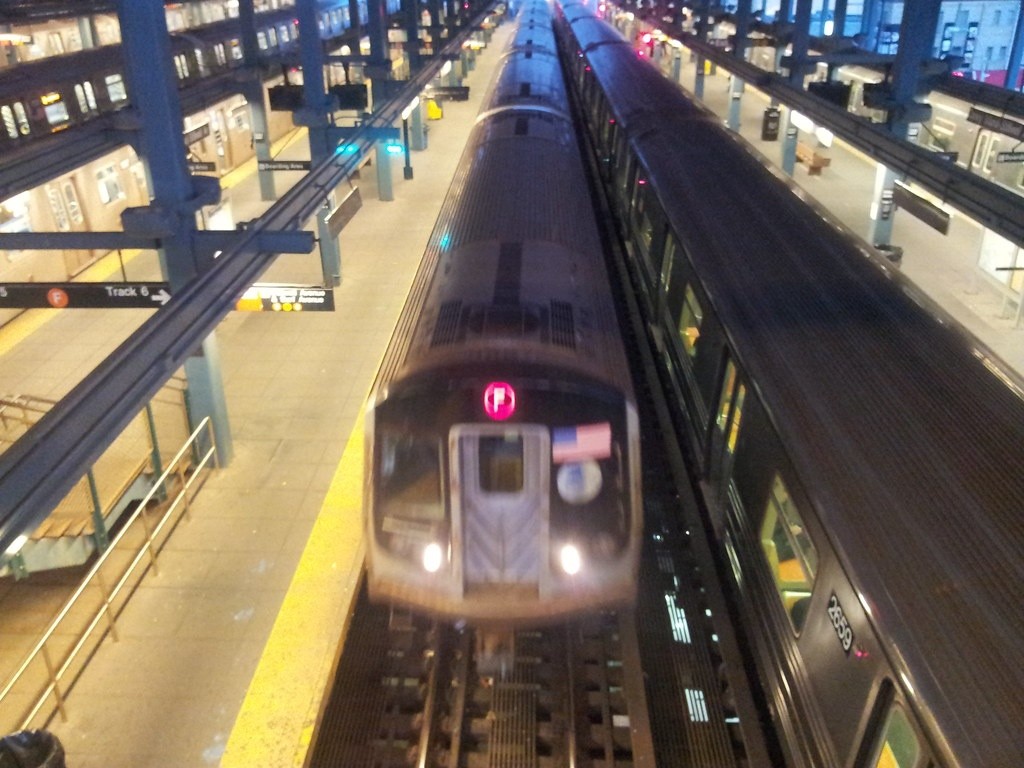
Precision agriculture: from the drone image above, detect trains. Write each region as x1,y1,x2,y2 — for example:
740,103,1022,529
361,0,644,631
548,0,1023,768
